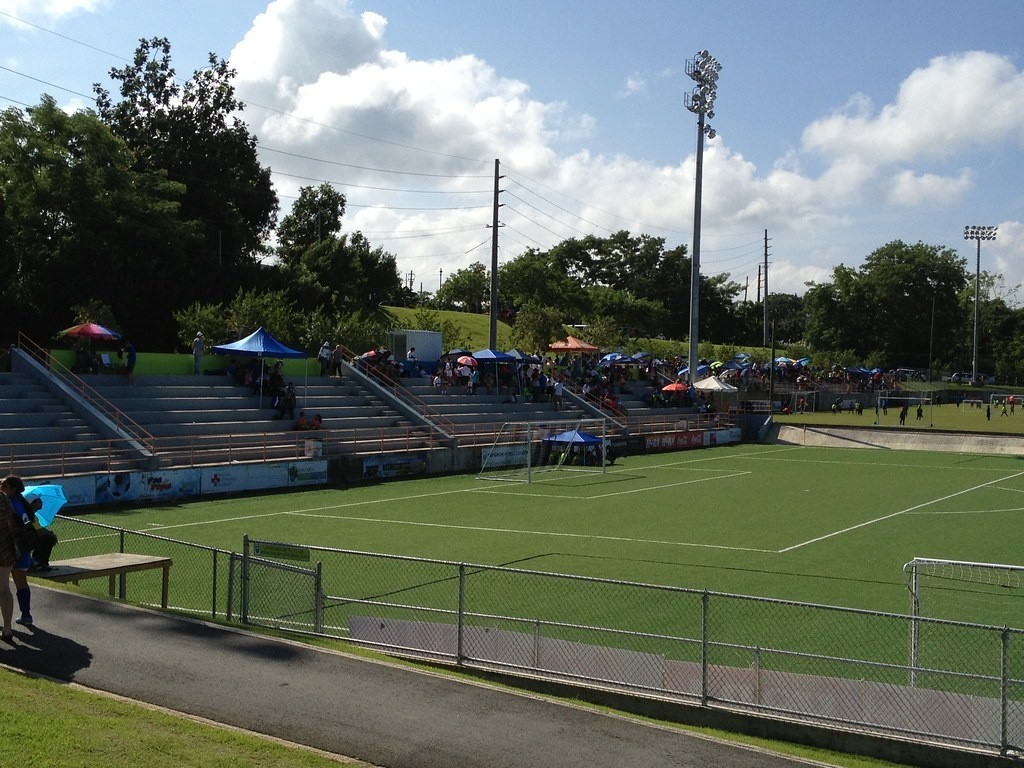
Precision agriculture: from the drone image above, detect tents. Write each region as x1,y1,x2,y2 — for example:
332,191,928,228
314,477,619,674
211,327,308,408
472,348,544,396
692,375,738,409
543,429,603,466
549,335,598,351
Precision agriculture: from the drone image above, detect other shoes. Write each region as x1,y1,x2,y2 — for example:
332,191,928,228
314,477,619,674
0,629,13,642
17,616,31,625
472,393,479,396
30,564,54,572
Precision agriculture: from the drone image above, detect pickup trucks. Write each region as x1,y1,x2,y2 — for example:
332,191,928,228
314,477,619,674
882,368,926,382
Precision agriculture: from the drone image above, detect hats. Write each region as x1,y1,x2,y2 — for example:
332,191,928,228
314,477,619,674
323,341,329,347
197,332,203,337
24,492,42,504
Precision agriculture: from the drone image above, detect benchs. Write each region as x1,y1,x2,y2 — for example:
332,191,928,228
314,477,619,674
0,364,894,468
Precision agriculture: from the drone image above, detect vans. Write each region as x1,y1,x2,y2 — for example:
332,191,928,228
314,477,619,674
951,372,996,385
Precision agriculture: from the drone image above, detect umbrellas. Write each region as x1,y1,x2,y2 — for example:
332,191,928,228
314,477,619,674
440,348,472,361
843,367,883,373
456,356,478,365
600,352,650,365
662,382,688,390
21,483,68,528
60,322,121,340
679,352,810,377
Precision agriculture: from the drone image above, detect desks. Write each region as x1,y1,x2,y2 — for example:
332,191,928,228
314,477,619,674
27,552,173,608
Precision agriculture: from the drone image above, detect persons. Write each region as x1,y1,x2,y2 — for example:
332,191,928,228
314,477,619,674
370,347,433,386
434,354,842,415
317,341,343,377
986,394,1014,421
0,477,58,641
848,400,863,415
191,332,206,375
227,359,322,430
547,444,616,467
956,398,960,408
847,376,886,393
910,370,921,381
547,352,580,366
125,341,137,386
882,399,923,425
936,394,942,406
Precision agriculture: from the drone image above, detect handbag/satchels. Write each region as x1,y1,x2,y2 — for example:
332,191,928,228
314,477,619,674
13,519,40,554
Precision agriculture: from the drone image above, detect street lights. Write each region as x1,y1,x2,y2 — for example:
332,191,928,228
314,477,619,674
964,226,998,382
683,49,723,384
